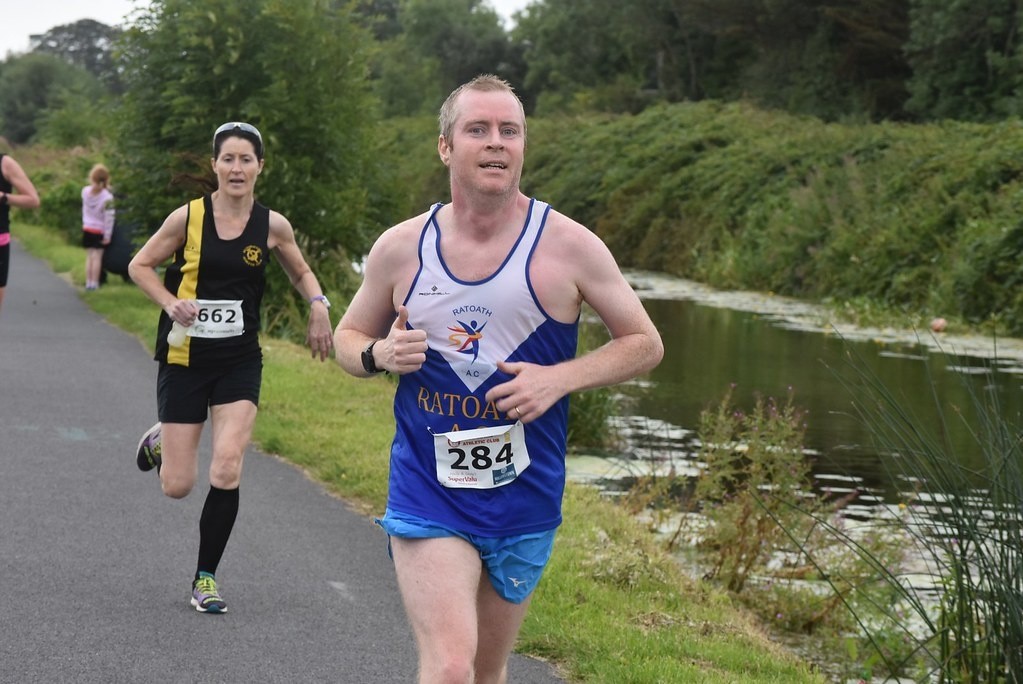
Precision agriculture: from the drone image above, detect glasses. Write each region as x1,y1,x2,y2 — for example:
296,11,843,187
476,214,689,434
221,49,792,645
213,122,263,152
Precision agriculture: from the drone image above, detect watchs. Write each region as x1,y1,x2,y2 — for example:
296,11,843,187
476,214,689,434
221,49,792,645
361,339,390,375
1,193,7,202
310,295,331,307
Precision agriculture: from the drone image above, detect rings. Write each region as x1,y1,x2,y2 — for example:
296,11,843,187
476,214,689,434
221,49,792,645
515,406,521,415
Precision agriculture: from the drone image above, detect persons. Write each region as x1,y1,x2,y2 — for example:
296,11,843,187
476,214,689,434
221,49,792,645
99,192,138,284
0,153,40,306
80,164,115,290
331,76,664,684
127,122,334,612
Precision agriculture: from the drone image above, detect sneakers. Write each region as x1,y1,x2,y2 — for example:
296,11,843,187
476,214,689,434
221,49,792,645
190,571,227,613
136,422,162,472
